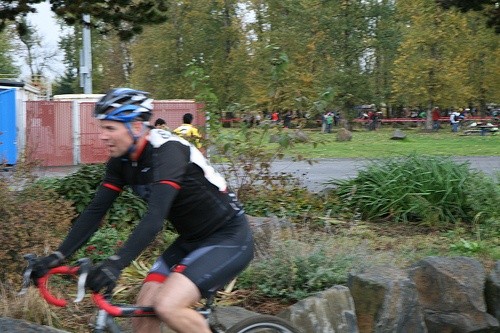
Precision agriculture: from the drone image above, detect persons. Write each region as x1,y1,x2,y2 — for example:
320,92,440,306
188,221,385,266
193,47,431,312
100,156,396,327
22,88,253,333
256,105,500,137
152,113,202,153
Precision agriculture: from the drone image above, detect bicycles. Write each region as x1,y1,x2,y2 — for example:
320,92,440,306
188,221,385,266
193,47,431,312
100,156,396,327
20,252,302,332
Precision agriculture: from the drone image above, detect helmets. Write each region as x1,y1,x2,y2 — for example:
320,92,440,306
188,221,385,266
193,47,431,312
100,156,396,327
92,87,154,122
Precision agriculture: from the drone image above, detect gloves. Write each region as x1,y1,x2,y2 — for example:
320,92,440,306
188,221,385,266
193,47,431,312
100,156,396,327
84,254,127,295
21,254,63,287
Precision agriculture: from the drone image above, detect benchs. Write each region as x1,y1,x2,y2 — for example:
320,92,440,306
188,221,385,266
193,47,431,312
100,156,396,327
478,126,500,135
